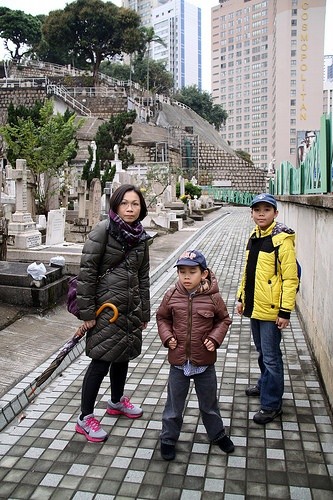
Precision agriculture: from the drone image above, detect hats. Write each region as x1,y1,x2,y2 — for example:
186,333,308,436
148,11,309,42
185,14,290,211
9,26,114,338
173,249,207,270
250,193,277,210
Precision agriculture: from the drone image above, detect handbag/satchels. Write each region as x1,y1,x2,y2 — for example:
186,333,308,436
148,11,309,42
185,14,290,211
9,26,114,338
67,276,98,319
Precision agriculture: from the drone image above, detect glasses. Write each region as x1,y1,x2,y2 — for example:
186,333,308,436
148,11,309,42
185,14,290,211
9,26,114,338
120,201,141,211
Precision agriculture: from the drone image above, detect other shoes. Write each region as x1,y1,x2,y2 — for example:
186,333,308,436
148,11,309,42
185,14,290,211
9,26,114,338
253,408,283,424
160,442,176,461
216,436,235,453
245,387,261,396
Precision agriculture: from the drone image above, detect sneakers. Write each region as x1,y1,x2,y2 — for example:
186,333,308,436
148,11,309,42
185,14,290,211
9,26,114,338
75,412,107,442
106,395,143,418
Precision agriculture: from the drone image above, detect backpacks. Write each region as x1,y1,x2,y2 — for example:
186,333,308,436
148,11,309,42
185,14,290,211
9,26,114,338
274,247,302,295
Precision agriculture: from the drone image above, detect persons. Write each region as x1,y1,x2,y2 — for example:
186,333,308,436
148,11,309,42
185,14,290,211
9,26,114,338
156,250,236,460
236,193,299,421
75,184,151,441
298,131,316,164
